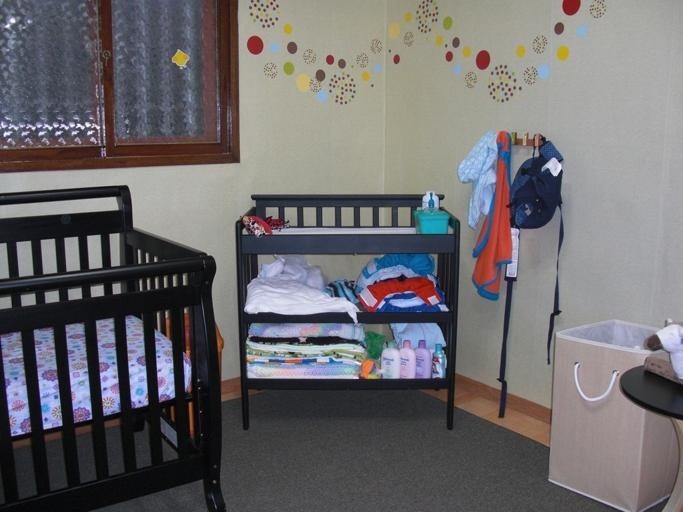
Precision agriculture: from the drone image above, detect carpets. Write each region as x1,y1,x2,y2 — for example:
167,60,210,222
0,389,681,512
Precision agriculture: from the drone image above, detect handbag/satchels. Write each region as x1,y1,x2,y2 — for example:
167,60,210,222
512,134,563,228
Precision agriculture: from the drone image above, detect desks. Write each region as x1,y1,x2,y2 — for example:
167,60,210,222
620,364,683,512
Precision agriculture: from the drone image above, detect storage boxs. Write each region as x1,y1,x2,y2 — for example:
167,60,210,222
543,317,682,512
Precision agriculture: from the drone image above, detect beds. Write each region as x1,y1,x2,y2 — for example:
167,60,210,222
0,184,228,512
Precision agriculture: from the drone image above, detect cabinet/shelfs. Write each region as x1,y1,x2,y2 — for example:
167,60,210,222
233,191,462,433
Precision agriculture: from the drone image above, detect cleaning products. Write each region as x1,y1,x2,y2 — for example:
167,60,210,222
423,190,440,208
